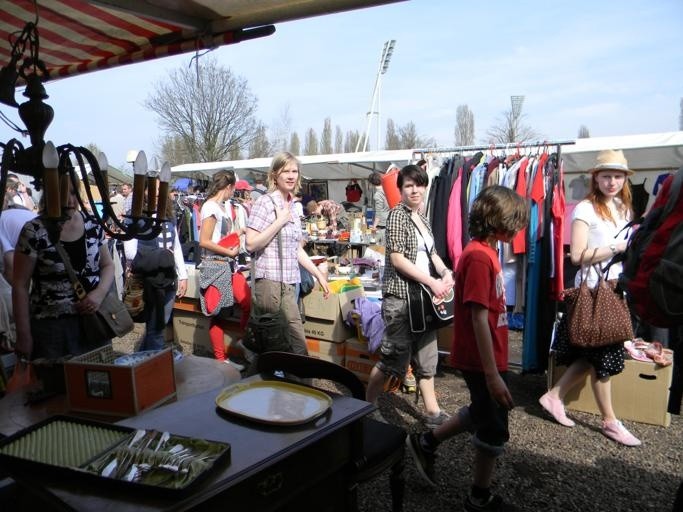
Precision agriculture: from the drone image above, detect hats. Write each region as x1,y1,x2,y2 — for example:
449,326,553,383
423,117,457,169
585,149,633,175
235,180,253,190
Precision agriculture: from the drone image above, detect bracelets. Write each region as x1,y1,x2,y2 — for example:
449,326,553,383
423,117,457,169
608,243,619,255
439,269,454,277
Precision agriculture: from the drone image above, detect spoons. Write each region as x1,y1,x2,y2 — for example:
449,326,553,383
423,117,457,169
100,427,215,483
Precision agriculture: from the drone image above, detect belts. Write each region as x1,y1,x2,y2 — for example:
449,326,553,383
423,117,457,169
376,226,385,229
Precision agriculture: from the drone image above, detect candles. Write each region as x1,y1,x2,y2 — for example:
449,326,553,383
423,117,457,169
132,151,148,217
147,155,159,212
41,138,63,218
98,153,111,202
156,160,171,220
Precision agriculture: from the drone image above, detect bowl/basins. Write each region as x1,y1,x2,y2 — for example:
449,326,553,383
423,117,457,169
359,277,375,287
337,266,351,274
317,233,326,239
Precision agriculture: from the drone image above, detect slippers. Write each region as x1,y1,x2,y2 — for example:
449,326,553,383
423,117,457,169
622,337,673,366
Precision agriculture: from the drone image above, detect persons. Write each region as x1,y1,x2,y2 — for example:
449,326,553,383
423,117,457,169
538,150,644,446
365,165,455,426
370,172,389,229
405,185,521,512
0,151,350,386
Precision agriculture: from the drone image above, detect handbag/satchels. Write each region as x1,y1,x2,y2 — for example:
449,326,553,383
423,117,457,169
242,311,291,353
87,293,135,341
0,353,18,392
298,262,314,292
563,247,633,349
406,269,457,333
122,276,145,317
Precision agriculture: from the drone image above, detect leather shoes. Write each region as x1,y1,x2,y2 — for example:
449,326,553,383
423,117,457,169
224,358,245,371
236,339,256,363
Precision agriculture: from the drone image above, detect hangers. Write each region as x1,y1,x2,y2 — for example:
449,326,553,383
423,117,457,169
416,139,551,158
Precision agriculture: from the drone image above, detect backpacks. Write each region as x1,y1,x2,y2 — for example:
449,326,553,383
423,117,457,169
603,172,682,329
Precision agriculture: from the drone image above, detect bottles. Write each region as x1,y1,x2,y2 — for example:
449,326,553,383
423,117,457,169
369,229,376,245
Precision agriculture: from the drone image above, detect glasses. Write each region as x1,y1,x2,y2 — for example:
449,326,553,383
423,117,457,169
224,170,234,175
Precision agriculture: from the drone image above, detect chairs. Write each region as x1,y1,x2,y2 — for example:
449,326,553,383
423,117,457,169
251,350,405,511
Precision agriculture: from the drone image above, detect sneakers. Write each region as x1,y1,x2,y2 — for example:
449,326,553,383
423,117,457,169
600,421,640,446
366,408,389,424
422,410,452,428
538,393,575,427
401,365,415,387
463,493,524,511
406,433,437,486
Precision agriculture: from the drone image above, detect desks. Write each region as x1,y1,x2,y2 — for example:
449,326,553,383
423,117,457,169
1,357,406,511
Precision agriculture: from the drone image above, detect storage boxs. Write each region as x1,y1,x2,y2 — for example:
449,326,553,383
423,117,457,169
553,348,674,426
62,342,177,418
171,264,416,388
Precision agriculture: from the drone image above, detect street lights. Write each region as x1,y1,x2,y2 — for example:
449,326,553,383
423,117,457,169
346,36,398,154
